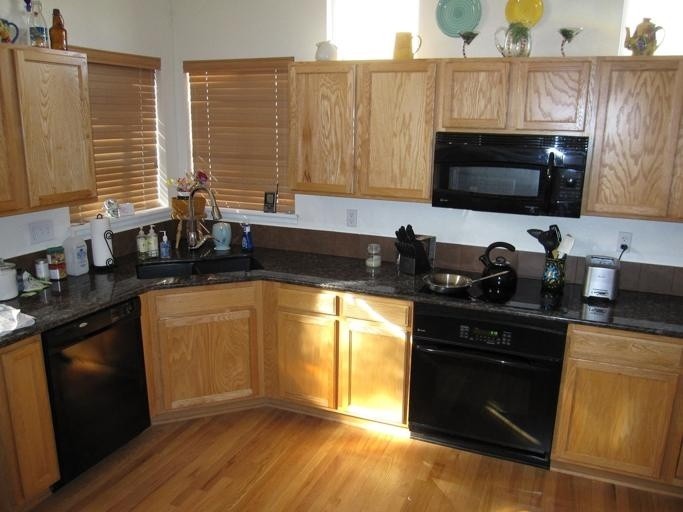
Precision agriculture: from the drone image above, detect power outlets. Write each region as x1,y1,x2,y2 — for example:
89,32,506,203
617,232,633,253
346,208,357,227
28,219,55,245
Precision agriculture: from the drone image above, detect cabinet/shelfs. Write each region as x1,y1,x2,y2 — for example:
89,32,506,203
274,281,411,441
287,58,438,204
152,281,267,426
441,56,592,131
0,332,61,512
0,42,98,217
580,55,683,223
549,323,683,499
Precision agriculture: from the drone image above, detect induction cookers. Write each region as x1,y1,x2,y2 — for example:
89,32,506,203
420,267,546,312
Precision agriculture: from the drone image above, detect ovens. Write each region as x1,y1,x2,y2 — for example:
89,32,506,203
408,340,563,470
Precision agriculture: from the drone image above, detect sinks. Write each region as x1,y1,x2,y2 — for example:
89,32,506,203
194,255,265,275
135,260,201,280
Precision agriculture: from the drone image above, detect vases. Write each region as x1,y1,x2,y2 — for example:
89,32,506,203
170,192,208,219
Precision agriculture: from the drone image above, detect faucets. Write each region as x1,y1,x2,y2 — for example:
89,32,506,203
185,186,223,250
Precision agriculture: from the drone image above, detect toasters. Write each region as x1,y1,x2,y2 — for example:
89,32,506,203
581,301,615,324
582,255,621,301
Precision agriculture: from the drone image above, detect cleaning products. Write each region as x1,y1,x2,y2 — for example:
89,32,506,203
241,215,253,252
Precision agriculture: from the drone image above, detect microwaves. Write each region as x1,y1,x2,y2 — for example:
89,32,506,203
429,131,588,218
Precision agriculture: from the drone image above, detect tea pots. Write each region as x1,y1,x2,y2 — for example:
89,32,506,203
480,241,518,292
624,17,664,55
492,22,533,61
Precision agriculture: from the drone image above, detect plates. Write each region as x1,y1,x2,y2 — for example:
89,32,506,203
505,0,544,30
423,274,473,293
436,1,482,38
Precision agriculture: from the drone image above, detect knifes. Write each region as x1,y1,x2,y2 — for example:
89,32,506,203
393,224,416,243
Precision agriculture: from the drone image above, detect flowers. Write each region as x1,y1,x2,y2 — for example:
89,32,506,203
163,167,209,192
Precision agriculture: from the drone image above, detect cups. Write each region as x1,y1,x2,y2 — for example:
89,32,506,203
394,31,422,60
1,20,20,44
315,42,339,61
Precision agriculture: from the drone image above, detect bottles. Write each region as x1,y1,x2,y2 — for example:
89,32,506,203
366,268,382,281
365,244,382,268
39,274,90,304
35,246,66,281
27,2,70,50
541,291,564,312
241,223,254,251
541,256,565,289
212,222,232,250
0,258,18,302
